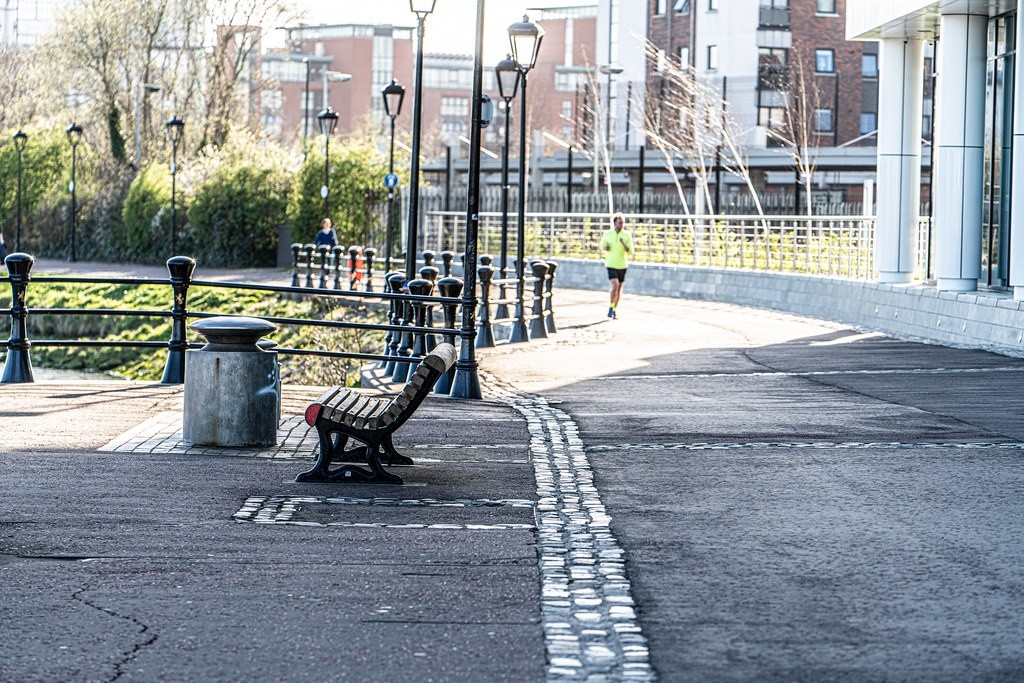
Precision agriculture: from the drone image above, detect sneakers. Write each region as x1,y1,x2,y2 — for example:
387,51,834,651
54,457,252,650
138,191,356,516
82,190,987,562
608,307,617,319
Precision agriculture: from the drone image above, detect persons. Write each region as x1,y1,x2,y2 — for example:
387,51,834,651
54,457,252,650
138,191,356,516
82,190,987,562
599,217,634,318
314,218,339,274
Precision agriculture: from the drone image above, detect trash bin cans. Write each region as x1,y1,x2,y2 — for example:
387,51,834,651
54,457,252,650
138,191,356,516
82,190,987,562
183,317,282,447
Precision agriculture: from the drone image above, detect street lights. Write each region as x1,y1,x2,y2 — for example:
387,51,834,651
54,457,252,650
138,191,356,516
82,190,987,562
66,123,85,262
381,77,406,272
504,13,547,320
165,113,186,258
496,52,522,320
403,0,438,278
318,106,341,219
13,130,28,253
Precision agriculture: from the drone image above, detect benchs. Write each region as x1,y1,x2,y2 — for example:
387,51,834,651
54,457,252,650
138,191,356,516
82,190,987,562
295,342,457,485
